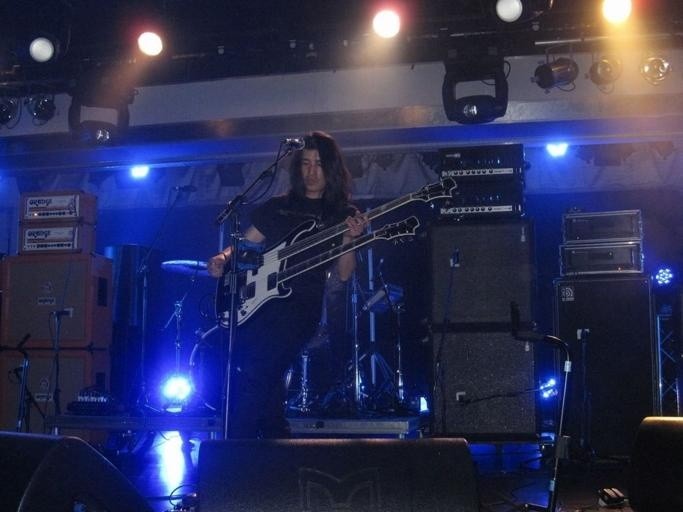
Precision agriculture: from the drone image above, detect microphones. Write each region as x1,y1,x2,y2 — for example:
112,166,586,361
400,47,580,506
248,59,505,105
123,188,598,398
510,301,520,334
283,136,306,151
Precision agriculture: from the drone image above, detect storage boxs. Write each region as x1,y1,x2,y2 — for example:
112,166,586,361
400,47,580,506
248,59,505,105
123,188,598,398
18,225,97,251
19,188,97,224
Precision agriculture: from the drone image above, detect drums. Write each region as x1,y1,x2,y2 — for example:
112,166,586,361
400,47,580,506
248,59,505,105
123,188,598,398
189,317,293,415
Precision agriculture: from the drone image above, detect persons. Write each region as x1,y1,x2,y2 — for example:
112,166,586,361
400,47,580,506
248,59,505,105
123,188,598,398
207,130,371,439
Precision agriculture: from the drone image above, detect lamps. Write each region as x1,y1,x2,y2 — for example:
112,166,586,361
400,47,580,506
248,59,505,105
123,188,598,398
0,68,60,128
216,161,244,186
441,36,515,125
59,67,141,149
531,32,677,94
345,155,363,179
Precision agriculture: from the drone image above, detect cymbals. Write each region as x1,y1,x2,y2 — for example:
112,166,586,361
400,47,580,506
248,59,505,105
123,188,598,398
161,261,212,277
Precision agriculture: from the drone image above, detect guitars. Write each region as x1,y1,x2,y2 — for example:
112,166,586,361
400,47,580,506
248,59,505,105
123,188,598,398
216,179,457,328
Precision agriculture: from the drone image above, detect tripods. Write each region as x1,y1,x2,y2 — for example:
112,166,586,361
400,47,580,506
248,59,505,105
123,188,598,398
285,207,398,417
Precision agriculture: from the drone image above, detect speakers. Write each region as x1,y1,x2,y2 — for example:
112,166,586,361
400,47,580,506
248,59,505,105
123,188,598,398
195,438,483,511
553,278,663,436
2,253,115,350
627,416,683,512
423,220,536,331
429,328,539,437
0,430,154,512
0,349,112,443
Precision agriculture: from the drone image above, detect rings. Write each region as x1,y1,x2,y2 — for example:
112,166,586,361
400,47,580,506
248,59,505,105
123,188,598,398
211,262,215,265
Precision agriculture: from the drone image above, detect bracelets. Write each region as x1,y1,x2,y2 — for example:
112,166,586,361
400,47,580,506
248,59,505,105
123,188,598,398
217,252,227,261
